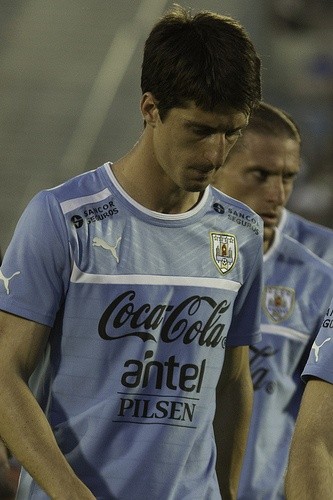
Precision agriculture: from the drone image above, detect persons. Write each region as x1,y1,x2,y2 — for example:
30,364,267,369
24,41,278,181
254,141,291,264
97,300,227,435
0,2,268,500
277,206,333,267
211,102,332,500
